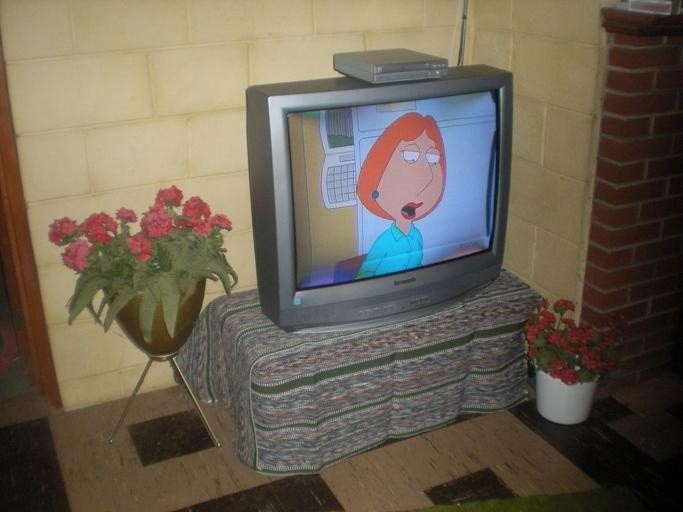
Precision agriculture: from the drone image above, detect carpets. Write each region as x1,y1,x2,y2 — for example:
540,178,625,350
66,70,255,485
406,487,644,511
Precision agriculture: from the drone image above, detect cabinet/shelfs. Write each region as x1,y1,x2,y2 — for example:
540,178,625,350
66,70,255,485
171,267,544,478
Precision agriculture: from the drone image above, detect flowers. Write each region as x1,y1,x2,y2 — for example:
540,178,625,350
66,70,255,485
523,298,632,384
45,185,238,345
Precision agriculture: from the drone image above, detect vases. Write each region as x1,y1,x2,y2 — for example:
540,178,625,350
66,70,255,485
533,354,599,427
102,277,205,362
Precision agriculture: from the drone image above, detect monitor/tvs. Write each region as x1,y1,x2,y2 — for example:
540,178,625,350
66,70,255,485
245,64,514,334
